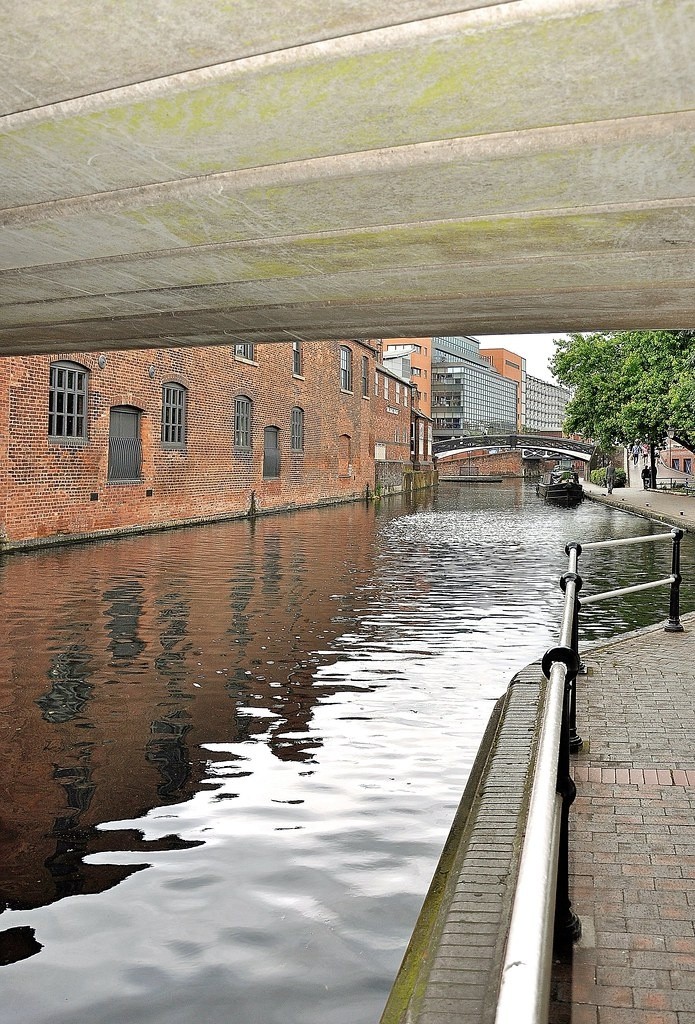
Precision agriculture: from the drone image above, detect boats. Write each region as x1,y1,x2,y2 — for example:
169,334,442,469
537,469,584,506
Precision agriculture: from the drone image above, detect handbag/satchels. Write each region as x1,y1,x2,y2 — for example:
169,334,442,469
631,453,633,460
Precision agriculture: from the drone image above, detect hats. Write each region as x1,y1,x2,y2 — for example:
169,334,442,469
609,461,614,464
644,444,647,448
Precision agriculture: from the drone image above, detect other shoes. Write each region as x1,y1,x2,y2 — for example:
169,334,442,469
610,491,612,494
608,489,611,493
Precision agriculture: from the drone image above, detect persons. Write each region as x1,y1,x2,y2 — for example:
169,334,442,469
641,442,649,466
632,441,639,465
605,459,618,495
571,465,575,472
641,465,657,489
